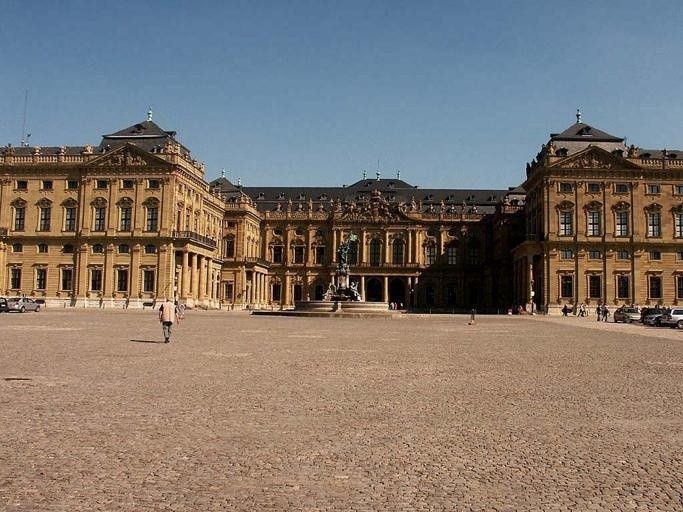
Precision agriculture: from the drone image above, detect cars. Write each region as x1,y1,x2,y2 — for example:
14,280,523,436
7,296,41,313
613,306,641,324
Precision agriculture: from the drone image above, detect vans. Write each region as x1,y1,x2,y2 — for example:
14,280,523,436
1,297,8,313
640,304,683,329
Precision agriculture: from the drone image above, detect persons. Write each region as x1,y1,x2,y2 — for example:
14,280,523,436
159,298,179,343
338,241,350,268
562,301,672,322
390,302,403,310
350,281,360,301
